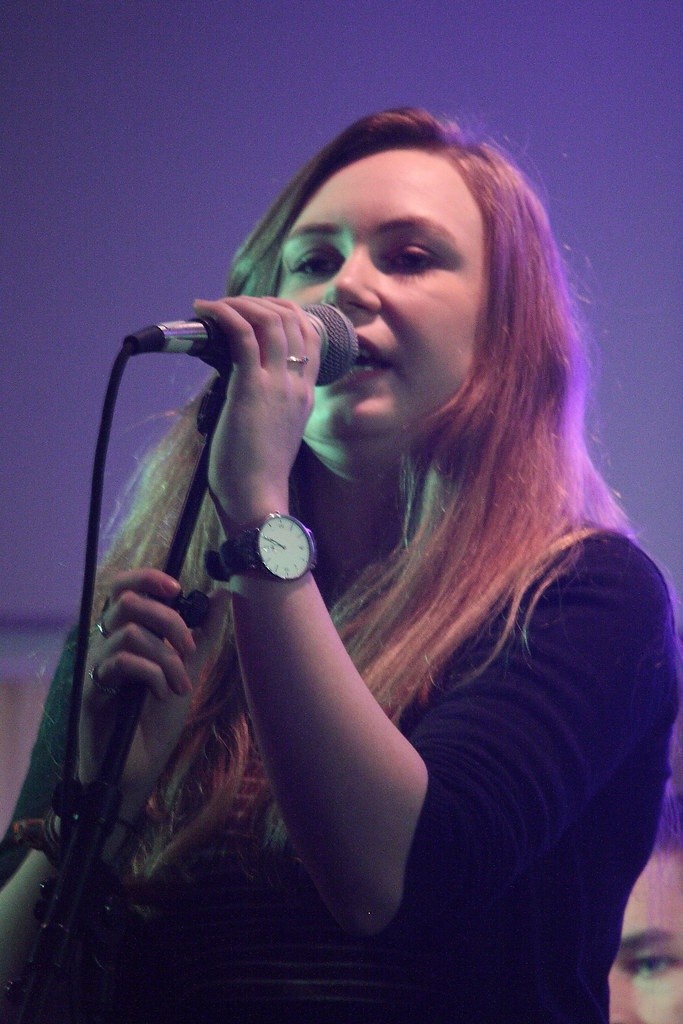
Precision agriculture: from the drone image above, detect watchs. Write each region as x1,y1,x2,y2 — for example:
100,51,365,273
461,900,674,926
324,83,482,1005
204,511,321,582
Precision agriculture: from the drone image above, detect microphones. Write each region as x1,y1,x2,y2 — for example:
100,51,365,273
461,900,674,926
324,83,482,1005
124,304,358,385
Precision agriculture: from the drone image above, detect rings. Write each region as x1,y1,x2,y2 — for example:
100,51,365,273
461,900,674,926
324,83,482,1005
96,617,109,639
287,357,309,364
87,662,119,695
100,596,109,613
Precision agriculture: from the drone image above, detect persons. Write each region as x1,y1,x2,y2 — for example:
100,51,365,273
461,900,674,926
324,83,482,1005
0,105,683,1024
